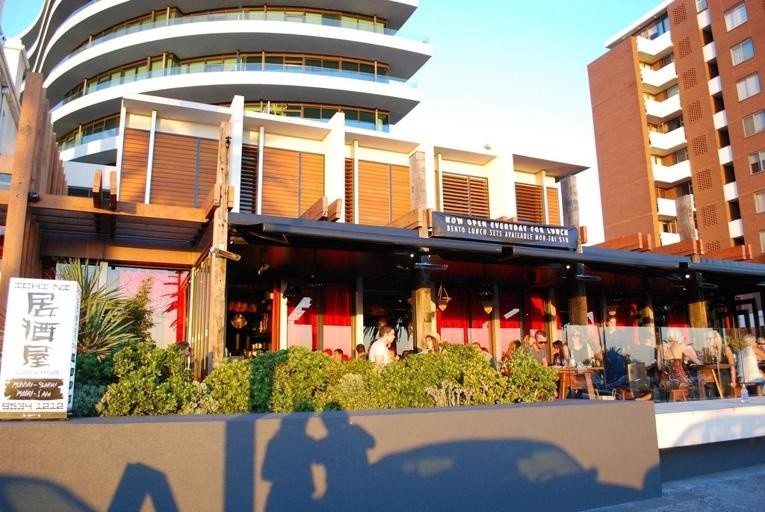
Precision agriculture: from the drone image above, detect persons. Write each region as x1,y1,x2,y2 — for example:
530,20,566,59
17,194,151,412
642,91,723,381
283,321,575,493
312,320,438,372
502,328,593,374
176,341,193,360
473,341,497,370
606,318,765,401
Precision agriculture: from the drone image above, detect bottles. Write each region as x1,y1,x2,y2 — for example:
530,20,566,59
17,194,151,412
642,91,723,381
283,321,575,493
703,347,719,365
590,356,601,367
739,384,749,404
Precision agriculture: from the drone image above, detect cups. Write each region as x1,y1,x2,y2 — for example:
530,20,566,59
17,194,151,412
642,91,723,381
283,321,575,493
555,359,560,366
567,356,577,369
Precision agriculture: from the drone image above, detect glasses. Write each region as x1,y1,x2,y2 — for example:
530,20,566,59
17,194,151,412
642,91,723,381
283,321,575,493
539,341,547,343
